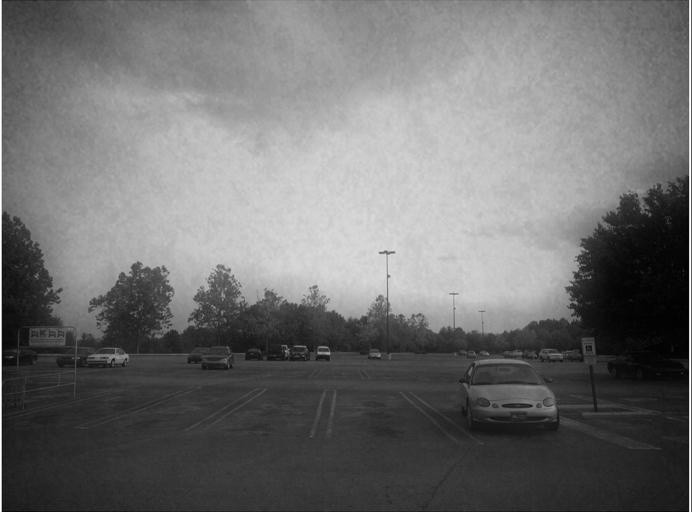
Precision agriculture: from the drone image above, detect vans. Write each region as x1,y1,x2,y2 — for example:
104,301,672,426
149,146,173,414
316,345,332,361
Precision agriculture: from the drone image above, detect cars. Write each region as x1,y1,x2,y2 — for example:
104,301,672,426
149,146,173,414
185,343,309,370
86,346,131,368
2,345,40,367
459,347,585,363
455,357,564,434
367,348,383,360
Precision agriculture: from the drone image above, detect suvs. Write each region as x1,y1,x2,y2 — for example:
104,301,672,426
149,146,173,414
55,346,97,367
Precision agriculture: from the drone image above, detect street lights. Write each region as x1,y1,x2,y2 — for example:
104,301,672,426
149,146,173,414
378,249,398,354
447,291,460,353
477,309,487,337
